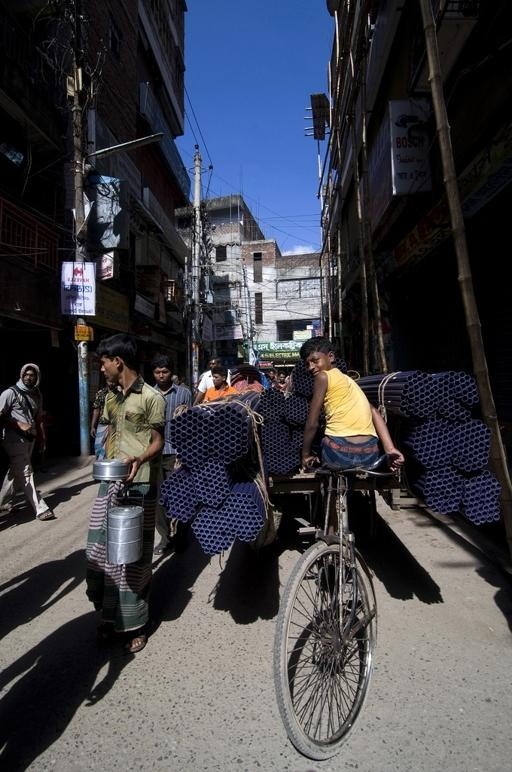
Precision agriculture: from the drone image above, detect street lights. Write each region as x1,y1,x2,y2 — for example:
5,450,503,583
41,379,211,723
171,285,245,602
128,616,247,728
69,131,167,457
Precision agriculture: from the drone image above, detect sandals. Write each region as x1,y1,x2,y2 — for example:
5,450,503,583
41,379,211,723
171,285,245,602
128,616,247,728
36,510,54,520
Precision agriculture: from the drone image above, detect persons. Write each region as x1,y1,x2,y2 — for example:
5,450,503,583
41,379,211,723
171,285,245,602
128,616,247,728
0,363,56,519
90,354,293,553
300,335,405,592
84,331,169,655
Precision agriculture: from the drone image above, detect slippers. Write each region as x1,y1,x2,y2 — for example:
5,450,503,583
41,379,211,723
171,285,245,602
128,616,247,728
126,634,146,654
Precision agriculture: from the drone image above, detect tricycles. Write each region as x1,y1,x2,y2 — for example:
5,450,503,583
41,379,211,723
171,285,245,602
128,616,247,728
271,453,407,761
